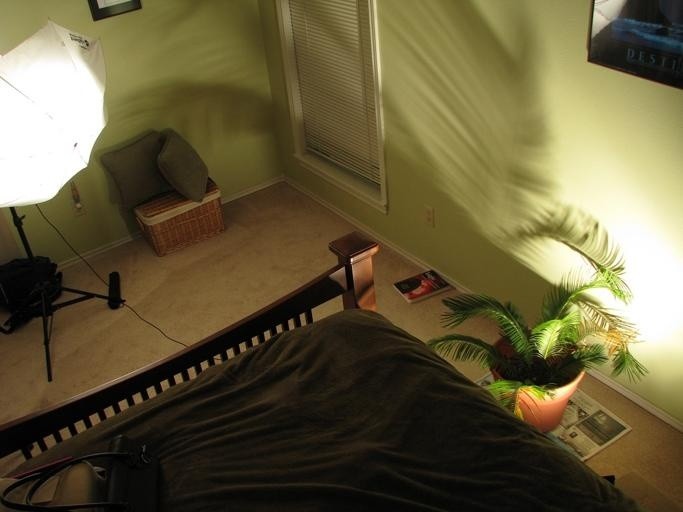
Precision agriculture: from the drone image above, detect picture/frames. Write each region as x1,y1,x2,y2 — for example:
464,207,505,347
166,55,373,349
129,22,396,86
88,0,142,22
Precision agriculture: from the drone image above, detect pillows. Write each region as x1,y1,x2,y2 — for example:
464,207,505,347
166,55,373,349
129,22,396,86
102,132,175,211
156,129,208,202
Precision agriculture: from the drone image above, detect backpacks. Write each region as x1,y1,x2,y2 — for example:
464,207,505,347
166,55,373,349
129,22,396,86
0,255,62,315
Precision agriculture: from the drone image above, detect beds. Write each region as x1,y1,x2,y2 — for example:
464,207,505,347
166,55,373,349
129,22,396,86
0,232,641,512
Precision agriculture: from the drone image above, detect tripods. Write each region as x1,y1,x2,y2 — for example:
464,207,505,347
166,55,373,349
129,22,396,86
0,207,108,381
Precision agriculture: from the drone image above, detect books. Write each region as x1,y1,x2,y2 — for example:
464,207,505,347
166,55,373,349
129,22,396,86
393,269,453,304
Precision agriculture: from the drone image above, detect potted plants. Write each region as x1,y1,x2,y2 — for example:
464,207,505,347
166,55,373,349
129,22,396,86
425,264,649,433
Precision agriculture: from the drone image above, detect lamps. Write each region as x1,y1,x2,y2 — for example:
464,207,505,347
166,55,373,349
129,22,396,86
0,16,110,208
